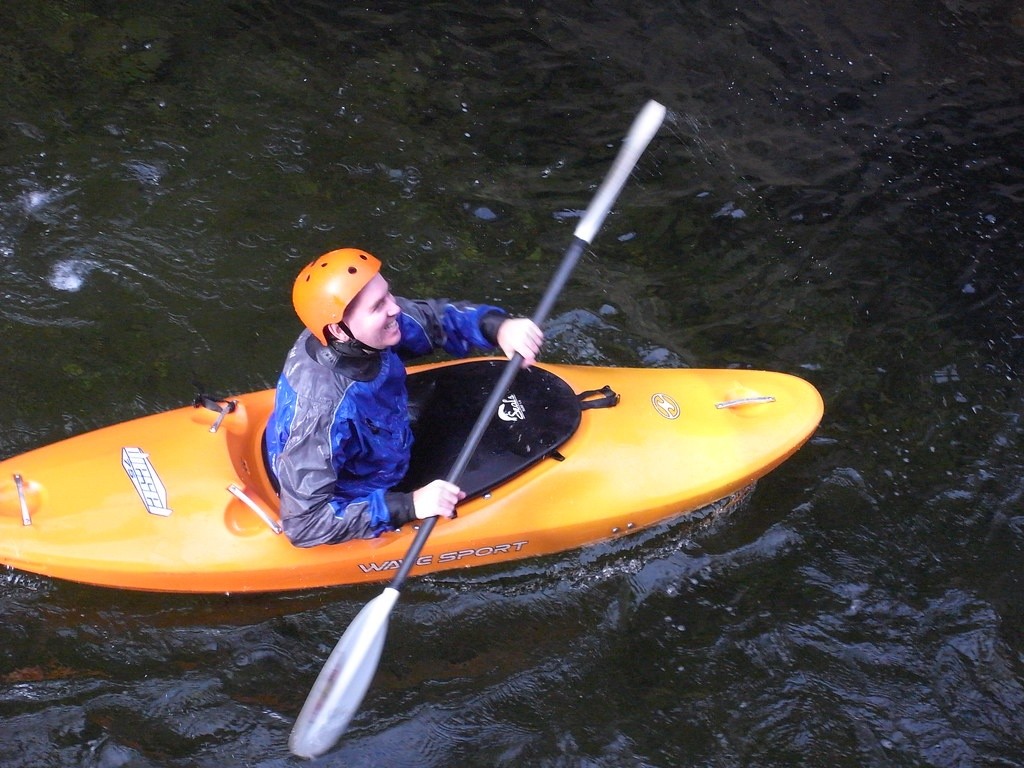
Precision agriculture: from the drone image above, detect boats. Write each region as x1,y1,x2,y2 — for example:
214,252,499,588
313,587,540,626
1,353,826,592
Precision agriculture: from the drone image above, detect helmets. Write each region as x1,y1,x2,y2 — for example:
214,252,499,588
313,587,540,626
293,249,381,346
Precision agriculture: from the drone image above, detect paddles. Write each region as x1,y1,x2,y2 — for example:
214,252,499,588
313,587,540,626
281,93,684,761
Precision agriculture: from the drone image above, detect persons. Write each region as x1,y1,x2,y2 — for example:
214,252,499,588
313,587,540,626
266,248,544,548
